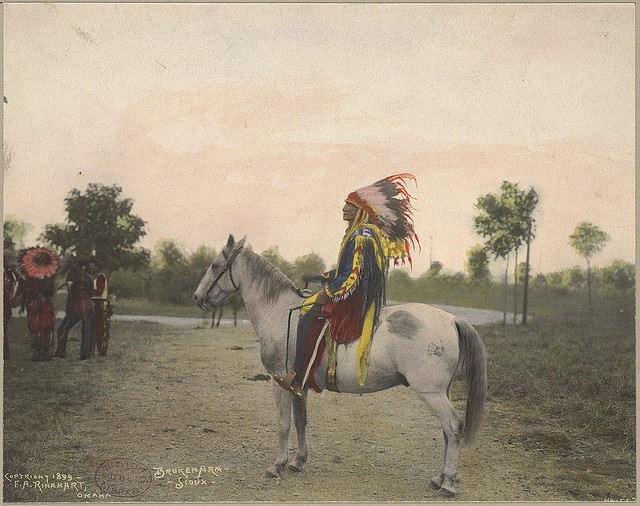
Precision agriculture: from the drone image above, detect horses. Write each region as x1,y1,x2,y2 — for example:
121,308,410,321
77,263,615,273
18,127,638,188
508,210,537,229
192,234,489,499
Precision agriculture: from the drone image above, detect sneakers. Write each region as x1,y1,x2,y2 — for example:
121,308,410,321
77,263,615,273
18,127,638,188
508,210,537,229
272,373,307,400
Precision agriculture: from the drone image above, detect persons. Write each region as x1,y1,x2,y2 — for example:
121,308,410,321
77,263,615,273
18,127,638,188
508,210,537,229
90,258,111,357
4,253,25,359
18,249,56,361
286,171,423,395
54,256,92,360
